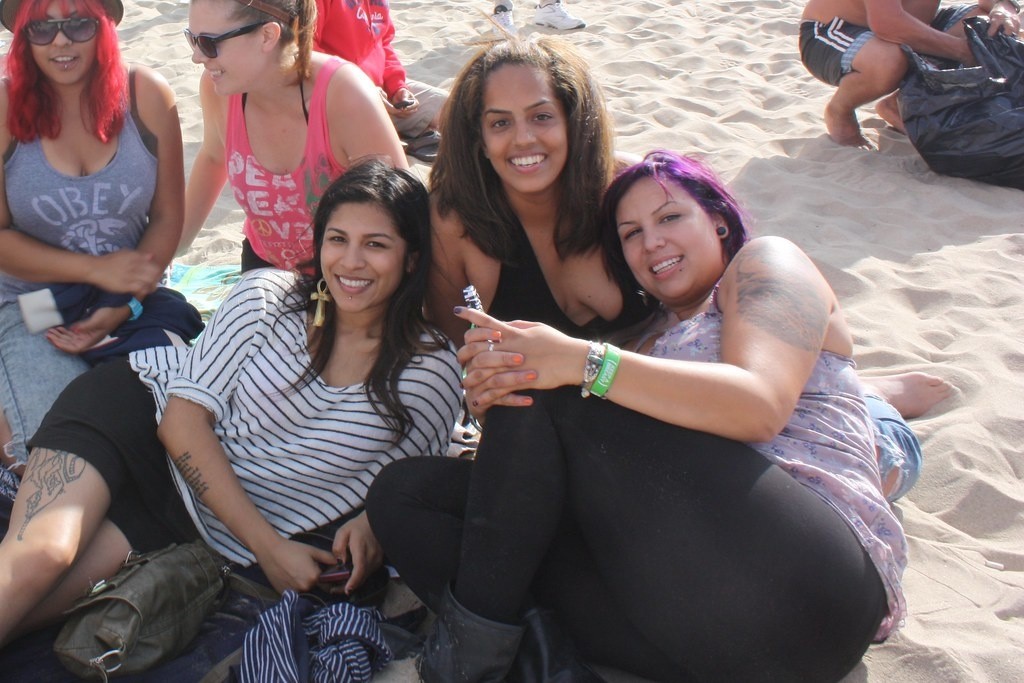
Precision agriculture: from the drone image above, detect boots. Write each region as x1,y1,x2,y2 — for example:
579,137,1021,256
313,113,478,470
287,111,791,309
377,577,529,683
507,609,607,683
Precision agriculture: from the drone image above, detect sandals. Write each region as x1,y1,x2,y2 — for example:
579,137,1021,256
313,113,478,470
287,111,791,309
398,130,442,162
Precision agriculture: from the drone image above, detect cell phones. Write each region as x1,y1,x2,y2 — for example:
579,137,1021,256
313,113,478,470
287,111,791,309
394,100,414,109
319,555,353,581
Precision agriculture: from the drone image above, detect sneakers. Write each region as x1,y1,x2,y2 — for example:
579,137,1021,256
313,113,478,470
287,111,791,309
534,0,586,30
490,0,515,38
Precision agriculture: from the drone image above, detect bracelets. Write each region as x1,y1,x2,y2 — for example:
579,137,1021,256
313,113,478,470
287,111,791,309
127,297,144,321
581,341,621,398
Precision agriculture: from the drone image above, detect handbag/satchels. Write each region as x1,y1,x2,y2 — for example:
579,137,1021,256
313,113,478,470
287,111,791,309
54,539,231,682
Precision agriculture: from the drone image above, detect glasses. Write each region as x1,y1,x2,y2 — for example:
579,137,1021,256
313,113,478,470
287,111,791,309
22,18,99,45
183,19,284,58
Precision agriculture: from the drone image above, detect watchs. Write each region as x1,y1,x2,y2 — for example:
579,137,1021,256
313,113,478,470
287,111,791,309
1000,0,1020,13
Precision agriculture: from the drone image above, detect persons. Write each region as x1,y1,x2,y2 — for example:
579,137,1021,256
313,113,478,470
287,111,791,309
423,32,962,504
367,151,909,683
493,0,586,34
313,0,450,161
0,0,185,476
0,162,463,649
172,1,409,275
798,0,1024,152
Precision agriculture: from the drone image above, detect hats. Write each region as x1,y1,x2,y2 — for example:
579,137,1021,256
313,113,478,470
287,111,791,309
0,0,124,34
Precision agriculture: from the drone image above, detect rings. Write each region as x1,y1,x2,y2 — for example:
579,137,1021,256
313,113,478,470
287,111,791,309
488,340,494,351
